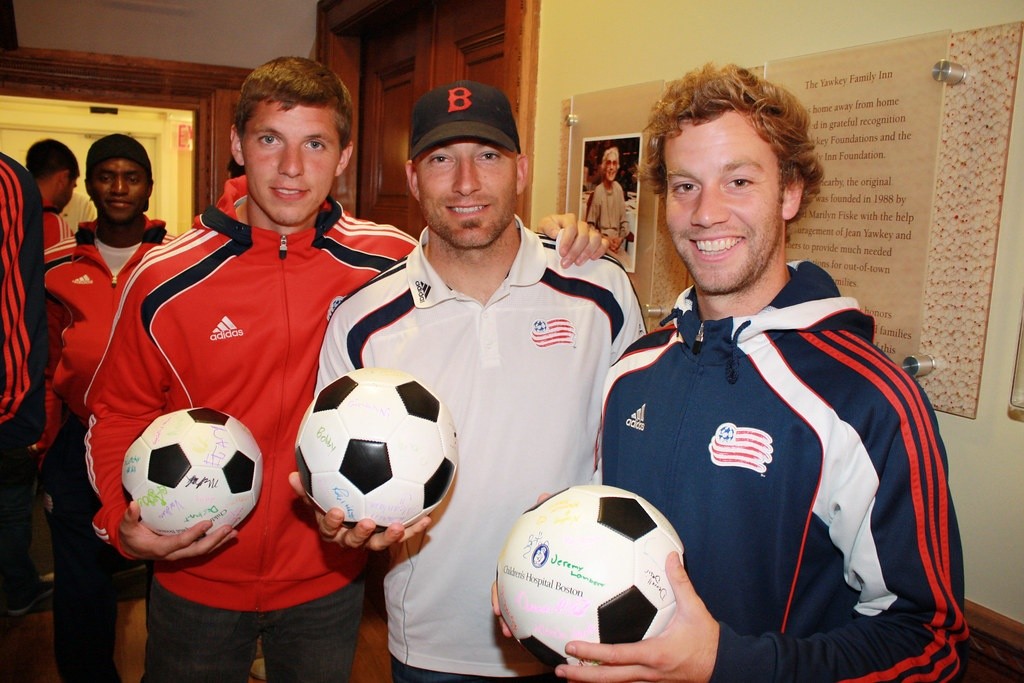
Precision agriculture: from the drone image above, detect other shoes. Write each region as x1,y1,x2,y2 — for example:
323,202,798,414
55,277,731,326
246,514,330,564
3,570,54,614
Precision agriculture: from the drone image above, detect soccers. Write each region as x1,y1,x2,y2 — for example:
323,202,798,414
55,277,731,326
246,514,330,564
495,484,688,667
123,404,265,536
295,370,458,531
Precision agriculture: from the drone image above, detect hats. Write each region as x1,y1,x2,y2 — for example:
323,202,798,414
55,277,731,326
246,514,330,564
409,80,521,156
86,134,152,211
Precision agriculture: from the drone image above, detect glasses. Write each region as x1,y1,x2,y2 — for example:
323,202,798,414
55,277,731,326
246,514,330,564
605,160,618,166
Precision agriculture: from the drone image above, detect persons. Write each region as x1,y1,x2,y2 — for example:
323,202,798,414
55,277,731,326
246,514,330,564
587,146,630,252
0,135,176,683
288,80,649,683
86,55,610,683
494,64,969,683
